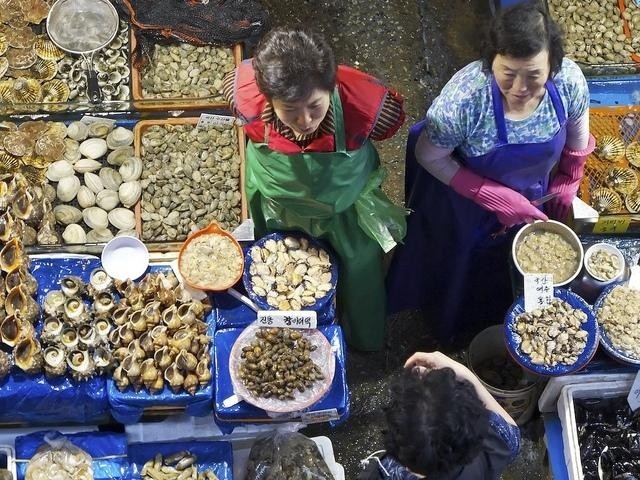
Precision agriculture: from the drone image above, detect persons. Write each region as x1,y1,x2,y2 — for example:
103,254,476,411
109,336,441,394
357,351,521,479
221,27,409,350
385,6,596,344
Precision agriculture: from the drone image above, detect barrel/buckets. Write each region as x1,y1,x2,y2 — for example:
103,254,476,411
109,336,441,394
466,323,545,427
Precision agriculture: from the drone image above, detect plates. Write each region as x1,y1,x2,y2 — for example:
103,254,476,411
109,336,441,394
583,244,625,282
501,287,600,376
241,231,339,318
590,273,640,366
228,317,337,414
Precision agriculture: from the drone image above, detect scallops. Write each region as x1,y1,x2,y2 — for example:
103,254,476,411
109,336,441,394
0,1,71,179
591,135,640,215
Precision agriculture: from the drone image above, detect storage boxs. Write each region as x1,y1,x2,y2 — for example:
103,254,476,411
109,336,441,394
536,0,640,73
557,378,640,480
132,117,249,241
129,16,244,117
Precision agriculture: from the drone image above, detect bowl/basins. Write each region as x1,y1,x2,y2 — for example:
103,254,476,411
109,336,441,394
100,236,150,281
509,218,584,289
178,223,245,292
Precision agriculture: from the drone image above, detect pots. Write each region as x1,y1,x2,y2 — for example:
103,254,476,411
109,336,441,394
46,0,120,104
